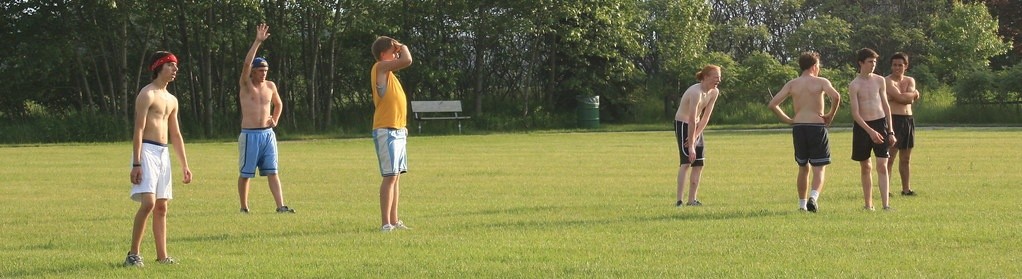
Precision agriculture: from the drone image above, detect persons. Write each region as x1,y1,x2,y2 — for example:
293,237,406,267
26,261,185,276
238,23,297,214
674,66,721,208
122,51,192,268
849,47,898,212
768,52,840,213
884,51,919,197
371,36,415,232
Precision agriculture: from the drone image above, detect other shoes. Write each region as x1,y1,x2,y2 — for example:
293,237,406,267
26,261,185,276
901,190,916,196
806,197,818,212
687,200,701,205
276,206,295,213
394,221,408,229
382,224,396,231
240,208,249,213
676,200,683,207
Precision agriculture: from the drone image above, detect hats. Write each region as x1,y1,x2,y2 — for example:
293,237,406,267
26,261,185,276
252,58,269,68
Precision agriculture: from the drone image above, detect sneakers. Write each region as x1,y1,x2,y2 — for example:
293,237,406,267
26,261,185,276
123,251,144,267
155,256,176,265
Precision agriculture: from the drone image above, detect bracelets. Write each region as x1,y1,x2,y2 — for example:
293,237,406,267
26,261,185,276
132,164,141,167
400,44,404,50
887,131,894,135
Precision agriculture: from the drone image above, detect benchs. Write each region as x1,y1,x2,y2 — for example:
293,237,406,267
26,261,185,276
411,100,470,136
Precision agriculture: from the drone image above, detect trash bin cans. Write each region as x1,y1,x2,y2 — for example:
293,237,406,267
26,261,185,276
576,94,600,129
